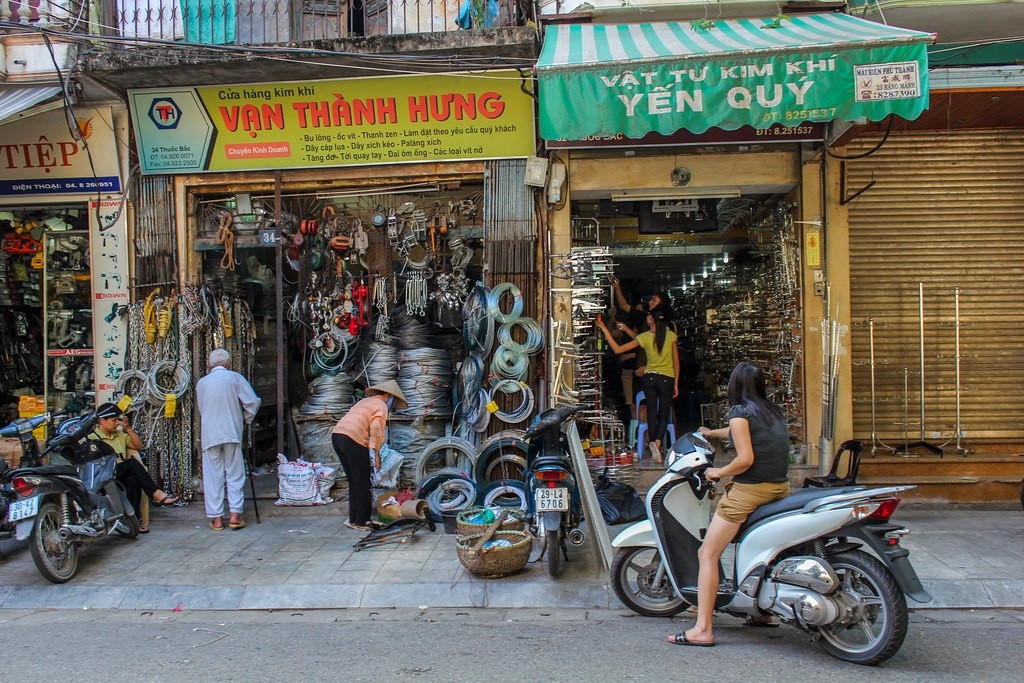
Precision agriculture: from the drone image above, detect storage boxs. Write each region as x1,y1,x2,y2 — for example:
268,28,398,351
19,412,46,440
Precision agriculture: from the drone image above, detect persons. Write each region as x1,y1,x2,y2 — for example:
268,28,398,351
667,362,788,646
87,402,179,533
595,275,679,464
331,379,409,528
195,348,261,530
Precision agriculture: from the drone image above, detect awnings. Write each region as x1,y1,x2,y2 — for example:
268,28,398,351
532,11,938,141
0,84,64,120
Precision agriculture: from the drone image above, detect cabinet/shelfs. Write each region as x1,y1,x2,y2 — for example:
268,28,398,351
42,230,95,459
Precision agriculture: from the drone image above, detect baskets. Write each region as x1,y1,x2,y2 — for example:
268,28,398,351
456,530,532,578
456,507,527,535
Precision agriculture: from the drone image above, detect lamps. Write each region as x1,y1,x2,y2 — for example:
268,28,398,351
611,187,744,201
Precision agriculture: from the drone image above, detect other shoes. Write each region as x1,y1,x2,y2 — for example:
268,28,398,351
153,494,180,506
351,519,383,528
139,525,150,533
649,442,663,463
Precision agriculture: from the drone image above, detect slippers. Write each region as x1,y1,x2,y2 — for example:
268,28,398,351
667,632,714,646
209,520,224,531
229,517,245,528
742,616,779,627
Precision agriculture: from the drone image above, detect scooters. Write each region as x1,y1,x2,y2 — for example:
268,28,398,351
519,401,595,578
610,426,933,666
0,401,143,583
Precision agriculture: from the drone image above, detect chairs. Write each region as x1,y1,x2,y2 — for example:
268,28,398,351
636,391,676,460
804,439,866,538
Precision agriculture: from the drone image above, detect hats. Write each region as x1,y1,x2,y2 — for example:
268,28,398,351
365,380,409,409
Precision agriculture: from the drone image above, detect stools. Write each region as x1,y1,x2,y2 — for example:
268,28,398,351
628,419,640,449
